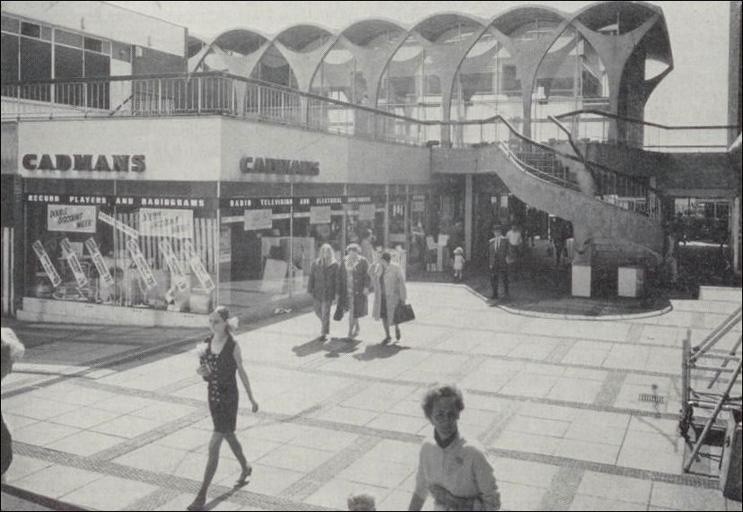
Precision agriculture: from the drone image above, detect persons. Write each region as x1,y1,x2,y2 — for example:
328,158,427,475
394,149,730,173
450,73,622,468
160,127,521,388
452,246,466,279
408,385,501,512
1,326,26,478
489,224,511,299
346,490,377,511
423,231,438,272
506,224,523,274
373,252,405,345
187,306,259,512
306,242,339,340
337,243,371,343
547,214,570,263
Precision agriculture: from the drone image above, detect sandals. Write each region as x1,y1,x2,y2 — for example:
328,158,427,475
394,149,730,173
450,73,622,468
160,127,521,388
185,497,205,512
233,467,252,483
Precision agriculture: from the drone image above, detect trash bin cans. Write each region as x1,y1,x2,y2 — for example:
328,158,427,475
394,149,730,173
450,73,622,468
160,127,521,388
617,265,645,298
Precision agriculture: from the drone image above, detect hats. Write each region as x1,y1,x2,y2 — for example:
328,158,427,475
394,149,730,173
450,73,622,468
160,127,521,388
381,252,392,259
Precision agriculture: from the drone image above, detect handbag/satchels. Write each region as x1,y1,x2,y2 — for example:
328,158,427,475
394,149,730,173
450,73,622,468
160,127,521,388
352,294,368,319
332,298,345,321
389,300,415,326
